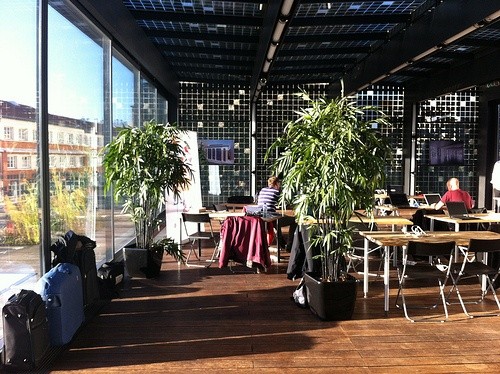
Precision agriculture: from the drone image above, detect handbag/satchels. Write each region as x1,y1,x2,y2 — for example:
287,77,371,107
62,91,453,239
96,261,124,291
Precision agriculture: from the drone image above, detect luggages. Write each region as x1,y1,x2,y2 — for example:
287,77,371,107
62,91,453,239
34,262,86,349
3,288,54,374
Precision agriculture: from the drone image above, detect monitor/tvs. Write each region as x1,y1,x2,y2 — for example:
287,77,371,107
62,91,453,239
198,139,234,164
421,140,464,166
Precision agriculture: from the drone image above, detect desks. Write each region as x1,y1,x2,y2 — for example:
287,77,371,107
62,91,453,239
298,218,413,265
424,213,500,264
359,231,500,311
229,209,293,217
197,213,280,263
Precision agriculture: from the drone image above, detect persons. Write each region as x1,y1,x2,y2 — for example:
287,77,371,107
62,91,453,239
258,177,297,251
435,178,473,215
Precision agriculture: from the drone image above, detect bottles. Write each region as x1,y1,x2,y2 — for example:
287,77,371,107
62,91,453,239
263,202,267,218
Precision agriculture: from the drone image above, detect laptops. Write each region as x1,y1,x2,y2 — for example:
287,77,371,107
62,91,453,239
446,201,481,221
388,194,418,210
422,193,446,208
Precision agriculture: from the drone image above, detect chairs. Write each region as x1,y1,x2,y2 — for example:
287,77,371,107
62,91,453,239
446,238,500,318
181,196,253,267
395,240,456,321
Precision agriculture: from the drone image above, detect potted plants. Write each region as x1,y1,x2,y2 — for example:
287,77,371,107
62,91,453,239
98,118,196,276
264,78,391,320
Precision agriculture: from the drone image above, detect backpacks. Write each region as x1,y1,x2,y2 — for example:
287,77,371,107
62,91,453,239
48,229,113,319
